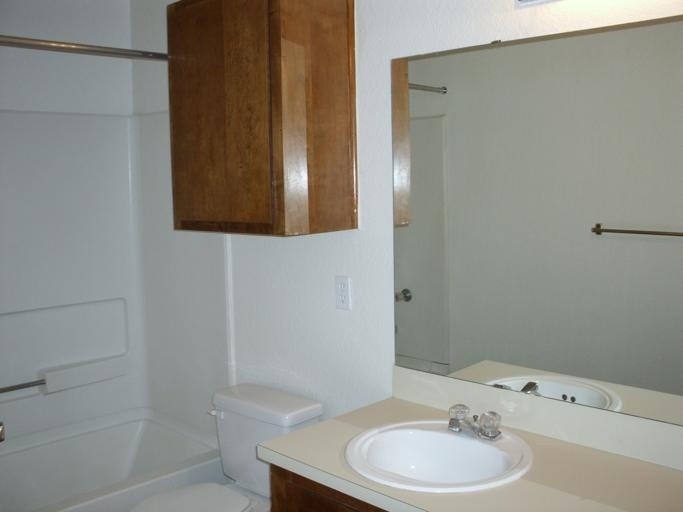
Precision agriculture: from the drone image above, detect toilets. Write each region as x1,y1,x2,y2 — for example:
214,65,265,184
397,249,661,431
134,386,322,512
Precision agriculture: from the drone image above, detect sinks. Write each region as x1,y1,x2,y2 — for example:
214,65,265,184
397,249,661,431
344,418,535,491
488,374,623,410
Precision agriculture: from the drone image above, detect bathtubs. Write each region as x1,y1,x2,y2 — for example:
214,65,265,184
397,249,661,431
0,410,221,512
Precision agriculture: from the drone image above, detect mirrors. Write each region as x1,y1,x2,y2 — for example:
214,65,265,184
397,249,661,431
391,14,683,429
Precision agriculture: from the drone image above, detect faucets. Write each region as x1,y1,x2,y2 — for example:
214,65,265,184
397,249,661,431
448,406,476,433
522,380,541,393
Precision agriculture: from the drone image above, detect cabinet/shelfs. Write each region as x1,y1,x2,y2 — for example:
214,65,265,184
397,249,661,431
391,58,412,227
166,0,359,236
